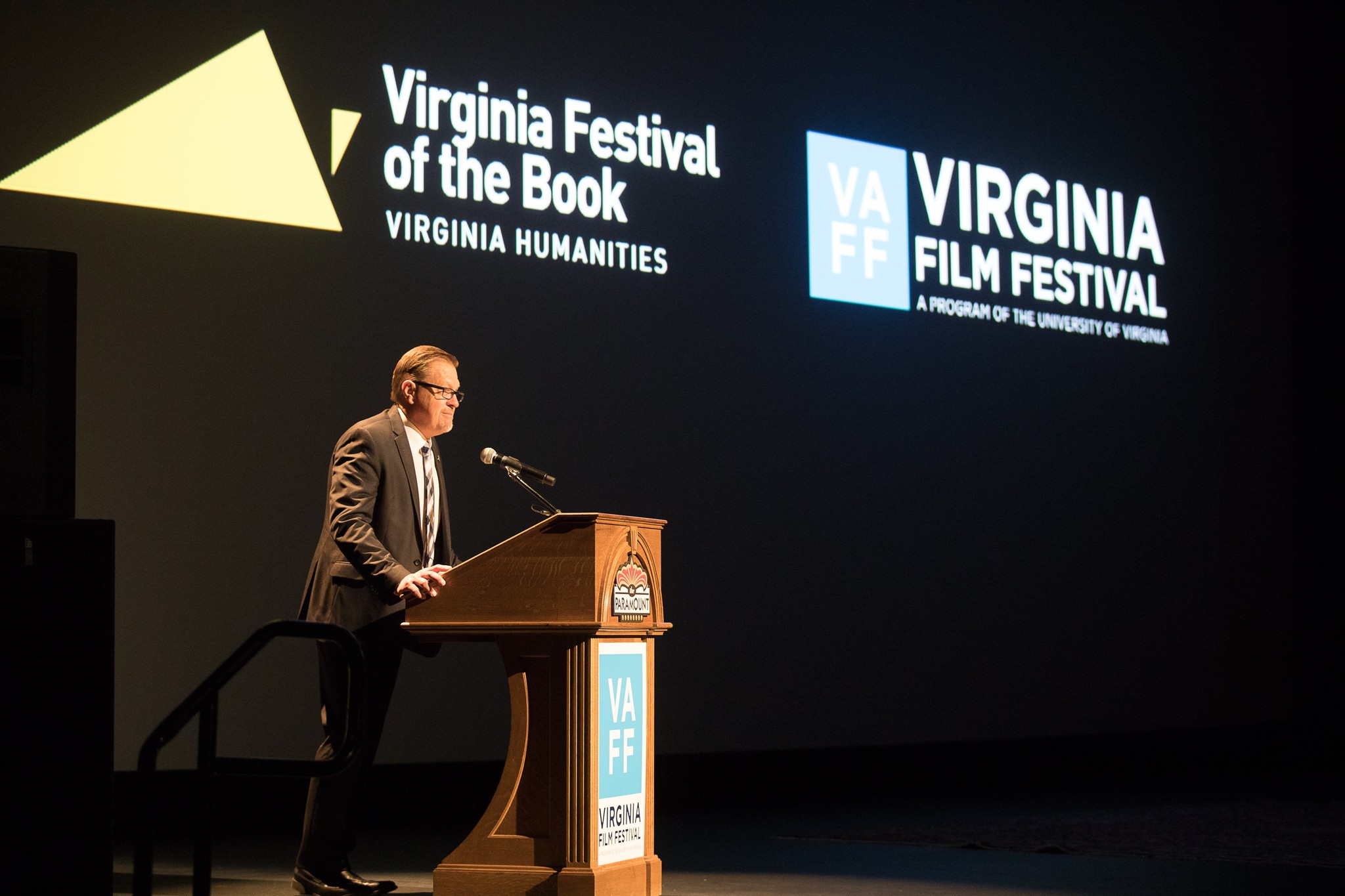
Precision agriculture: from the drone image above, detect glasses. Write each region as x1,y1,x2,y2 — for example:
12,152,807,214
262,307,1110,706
412,380,464,402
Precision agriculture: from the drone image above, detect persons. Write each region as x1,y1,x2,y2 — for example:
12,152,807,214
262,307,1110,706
290,346,464,896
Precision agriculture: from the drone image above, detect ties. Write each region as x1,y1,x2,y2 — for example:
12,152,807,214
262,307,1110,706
420,441,434,567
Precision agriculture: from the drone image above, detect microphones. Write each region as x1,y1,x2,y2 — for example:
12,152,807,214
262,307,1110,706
480,448,556,487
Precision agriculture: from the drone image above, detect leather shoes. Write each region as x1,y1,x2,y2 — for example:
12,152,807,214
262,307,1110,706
291,864,359,896
333,867,398,896
